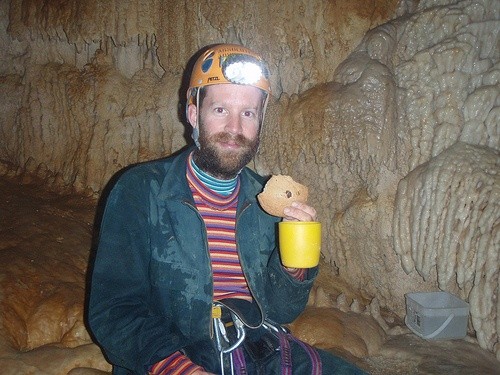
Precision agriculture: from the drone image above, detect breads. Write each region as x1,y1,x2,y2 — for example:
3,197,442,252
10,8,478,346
256,174,309,218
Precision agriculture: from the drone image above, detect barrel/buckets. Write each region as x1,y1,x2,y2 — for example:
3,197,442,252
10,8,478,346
404,291,470,341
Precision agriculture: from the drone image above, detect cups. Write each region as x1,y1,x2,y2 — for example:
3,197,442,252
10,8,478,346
279,220,322,269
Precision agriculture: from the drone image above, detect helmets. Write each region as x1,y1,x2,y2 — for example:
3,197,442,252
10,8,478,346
186,44,274,116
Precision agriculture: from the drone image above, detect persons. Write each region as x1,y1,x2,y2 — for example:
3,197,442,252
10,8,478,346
87,42,372,375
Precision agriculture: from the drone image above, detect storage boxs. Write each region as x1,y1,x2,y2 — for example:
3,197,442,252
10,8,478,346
405,291,470,340
